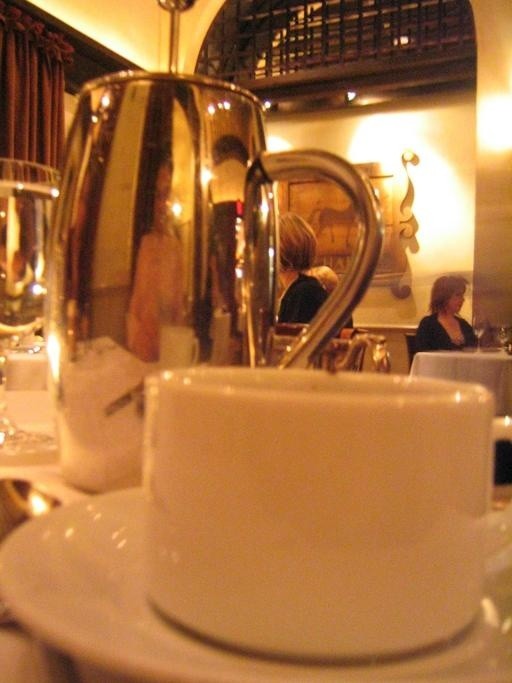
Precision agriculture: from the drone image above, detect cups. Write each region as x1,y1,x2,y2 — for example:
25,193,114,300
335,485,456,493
0,156,60,465
139,368,494,663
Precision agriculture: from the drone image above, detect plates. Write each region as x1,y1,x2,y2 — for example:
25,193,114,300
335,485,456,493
0,486,512,682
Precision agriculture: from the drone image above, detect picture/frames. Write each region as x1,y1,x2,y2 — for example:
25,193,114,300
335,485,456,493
276,150,419,298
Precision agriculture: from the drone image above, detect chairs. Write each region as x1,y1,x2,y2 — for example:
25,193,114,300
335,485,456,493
406,334,416,371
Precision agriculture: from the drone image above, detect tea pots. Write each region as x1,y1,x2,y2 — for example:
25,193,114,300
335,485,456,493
48,0,382,366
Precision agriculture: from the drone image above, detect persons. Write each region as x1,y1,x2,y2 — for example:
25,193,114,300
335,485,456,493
116,159,191,359
278,210,354,339
413,274,476,352
